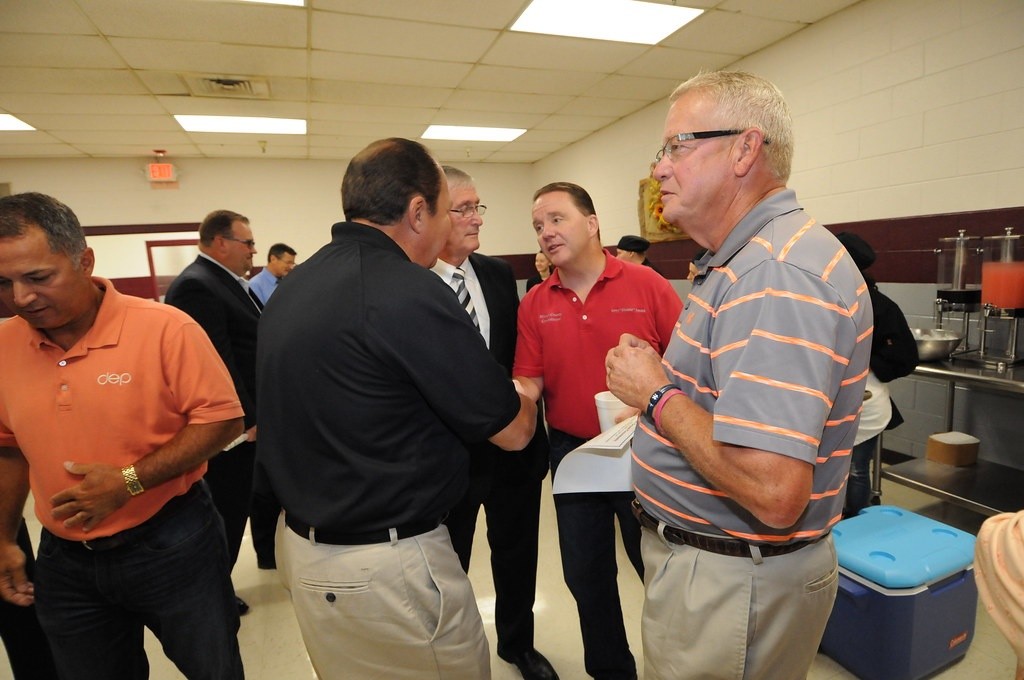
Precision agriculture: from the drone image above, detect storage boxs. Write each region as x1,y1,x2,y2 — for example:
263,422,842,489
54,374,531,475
928,431,981,466
820,505,977,680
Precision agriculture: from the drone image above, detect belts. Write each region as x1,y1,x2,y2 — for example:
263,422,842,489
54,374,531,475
629,499,833,559
285,509,448,545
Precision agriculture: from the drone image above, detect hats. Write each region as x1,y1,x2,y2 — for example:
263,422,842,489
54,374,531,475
834,231,877,270
617,235,650,253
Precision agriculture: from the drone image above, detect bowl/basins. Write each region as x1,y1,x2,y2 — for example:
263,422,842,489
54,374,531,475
909,328,966,363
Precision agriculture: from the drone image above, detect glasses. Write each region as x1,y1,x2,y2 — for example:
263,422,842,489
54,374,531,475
656,129,771,164
209,234,255,252
273,254,296,268
450,204,487,218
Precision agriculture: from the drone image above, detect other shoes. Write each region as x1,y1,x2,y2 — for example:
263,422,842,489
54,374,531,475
234,595,248,616
257,557,276,569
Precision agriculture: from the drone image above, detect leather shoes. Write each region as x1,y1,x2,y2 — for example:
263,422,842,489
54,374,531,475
496,642,560,680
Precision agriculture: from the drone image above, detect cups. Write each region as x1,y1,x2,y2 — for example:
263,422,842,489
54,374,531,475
593,390,628,434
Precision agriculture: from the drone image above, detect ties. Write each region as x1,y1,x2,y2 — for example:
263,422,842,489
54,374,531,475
452,267,481,333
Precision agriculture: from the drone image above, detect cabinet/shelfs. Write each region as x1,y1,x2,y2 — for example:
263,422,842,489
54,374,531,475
873,360,1024,516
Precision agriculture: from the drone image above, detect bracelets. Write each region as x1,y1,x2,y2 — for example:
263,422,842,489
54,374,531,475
653,389,686,434
645,384,682,425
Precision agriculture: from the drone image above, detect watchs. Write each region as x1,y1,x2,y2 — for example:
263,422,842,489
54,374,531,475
120,464,144,496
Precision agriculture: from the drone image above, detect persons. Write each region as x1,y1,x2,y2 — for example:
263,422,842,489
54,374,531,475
248,243,297,307
614,235,664,276
257,135,537,680
0,191,248,680
601,66,874,680
837,231,920,522
429,165,560,680
526,250,555,294
686,249,716,286
511,182,683,680
165,209,264,617
